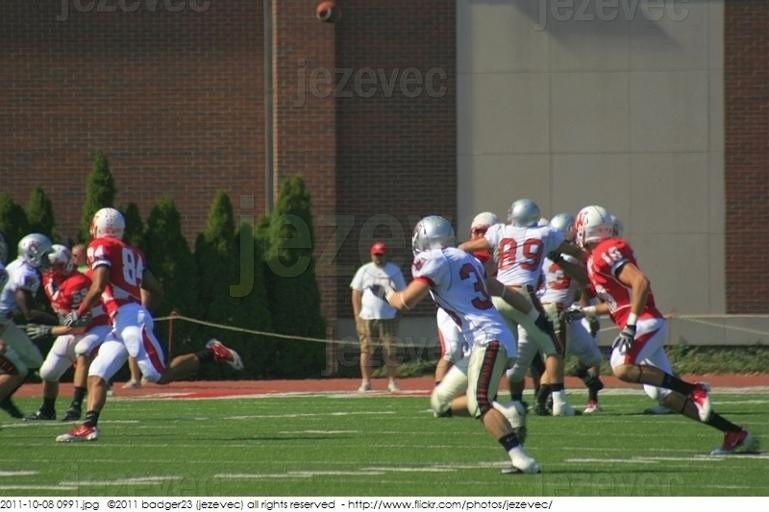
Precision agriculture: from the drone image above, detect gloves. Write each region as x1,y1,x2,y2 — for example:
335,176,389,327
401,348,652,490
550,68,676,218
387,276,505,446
368,283,394,304
560,303,585,324
587,317,600,338
27,323,51,340
612,325,637,352
64,309,78,328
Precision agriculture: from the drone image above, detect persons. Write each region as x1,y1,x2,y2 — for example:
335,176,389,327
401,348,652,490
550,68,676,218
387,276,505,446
2,208,245,443
348,199,750,476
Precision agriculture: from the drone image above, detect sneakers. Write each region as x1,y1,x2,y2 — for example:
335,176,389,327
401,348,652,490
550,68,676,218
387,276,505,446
510,395,527,410
570,364,585,376
205,338,244,371
644,404,671,413
552,389,575,415
502,444,539,473
22,408,56,421
710,428,749,454
494,400,528,446
62,401,82,419
122,380,140,389
358,383,370,392
56,424,98,442
536,384,563,416
387,381,400,392
584,400,598,413
0,399,22,418
689,383,712,422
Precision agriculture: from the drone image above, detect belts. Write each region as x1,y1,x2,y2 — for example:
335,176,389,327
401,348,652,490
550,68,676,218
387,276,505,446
507,285,522,288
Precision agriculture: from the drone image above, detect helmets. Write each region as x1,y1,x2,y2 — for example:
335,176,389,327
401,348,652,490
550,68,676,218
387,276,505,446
18,234,52,267
48,244,71,264
89,208,126,240
413,200,621,253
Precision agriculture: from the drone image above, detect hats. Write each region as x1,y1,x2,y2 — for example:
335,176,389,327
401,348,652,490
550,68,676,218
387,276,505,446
371,242,386,255
